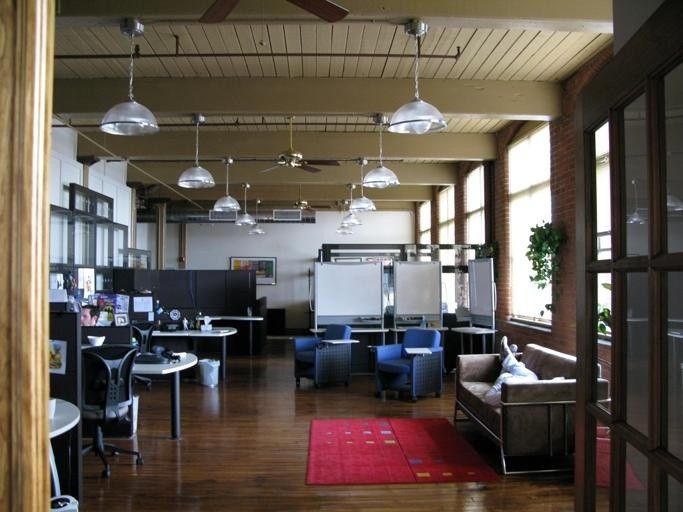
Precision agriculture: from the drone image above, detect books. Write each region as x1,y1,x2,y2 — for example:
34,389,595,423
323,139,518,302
211,329,228,333
47,268,131,321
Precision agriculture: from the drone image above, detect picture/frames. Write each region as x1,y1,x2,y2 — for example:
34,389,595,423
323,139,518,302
230,256,277,285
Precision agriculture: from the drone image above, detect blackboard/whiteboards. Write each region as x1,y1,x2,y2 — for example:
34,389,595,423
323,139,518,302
468,258,494,316
314,261,384,318
394,261,443,316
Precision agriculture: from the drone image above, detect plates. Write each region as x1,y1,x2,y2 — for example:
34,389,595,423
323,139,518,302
168,307,182,322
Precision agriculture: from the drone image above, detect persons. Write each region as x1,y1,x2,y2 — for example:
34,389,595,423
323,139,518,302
78,305,104,327
484,335,539,407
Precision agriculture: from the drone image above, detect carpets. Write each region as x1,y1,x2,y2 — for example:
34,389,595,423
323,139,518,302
306,418,499,485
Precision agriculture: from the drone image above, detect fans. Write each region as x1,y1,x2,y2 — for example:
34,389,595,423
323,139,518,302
198,0,350,24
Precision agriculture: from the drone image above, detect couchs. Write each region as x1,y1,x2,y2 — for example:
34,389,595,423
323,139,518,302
454,343,609,476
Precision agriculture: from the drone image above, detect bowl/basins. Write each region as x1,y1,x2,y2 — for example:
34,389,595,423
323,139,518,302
86,334,105,347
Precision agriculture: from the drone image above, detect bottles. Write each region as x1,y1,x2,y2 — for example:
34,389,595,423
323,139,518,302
182,314,200,330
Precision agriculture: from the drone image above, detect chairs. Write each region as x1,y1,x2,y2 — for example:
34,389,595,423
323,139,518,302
294,324,351,390
367,327,444,402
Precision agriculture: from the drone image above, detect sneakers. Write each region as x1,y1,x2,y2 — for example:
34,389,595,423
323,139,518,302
499,335,508,360
510,344,518,356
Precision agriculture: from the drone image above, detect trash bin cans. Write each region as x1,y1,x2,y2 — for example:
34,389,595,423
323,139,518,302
198,359,221,387
